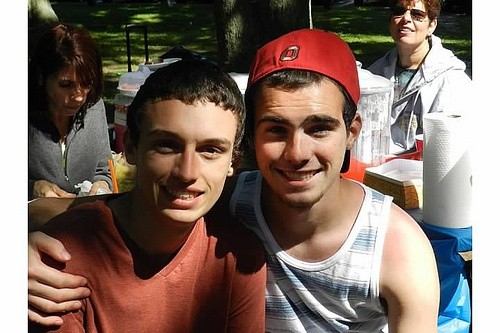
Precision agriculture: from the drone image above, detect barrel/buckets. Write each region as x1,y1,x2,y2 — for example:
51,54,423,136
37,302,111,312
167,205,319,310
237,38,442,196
351,60,393,166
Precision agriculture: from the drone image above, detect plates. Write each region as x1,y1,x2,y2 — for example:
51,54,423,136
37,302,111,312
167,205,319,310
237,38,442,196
118,85,138,97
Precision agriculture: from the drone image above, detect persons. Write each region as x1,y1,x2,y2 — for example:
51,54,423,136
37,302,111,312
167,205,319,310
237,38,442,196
366,0,472,155
28,23,113,198
28,29,440,333
28,59,266,333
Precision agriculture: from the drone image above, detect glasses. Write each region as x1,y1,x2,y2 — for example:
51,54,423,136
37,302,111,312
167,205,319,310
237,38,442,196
390,6,427,21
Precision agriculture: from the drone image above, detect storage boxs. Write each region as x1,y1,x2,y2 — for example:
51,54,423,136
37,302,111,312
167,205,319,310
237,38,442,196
364,158,423,210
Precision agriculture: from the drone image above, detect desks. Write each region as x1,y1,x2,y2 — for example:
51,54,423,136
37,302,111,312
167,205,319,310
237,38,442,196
400,208,472,333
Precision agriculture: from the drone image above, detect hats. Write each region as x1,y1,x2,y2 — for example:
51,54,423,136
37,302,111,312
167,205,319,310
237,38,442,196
244,29,359,174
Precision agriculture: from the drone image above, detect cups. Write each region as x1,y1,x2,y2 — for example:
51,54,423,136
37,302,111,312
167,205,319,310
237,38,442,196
414,135,423,160
